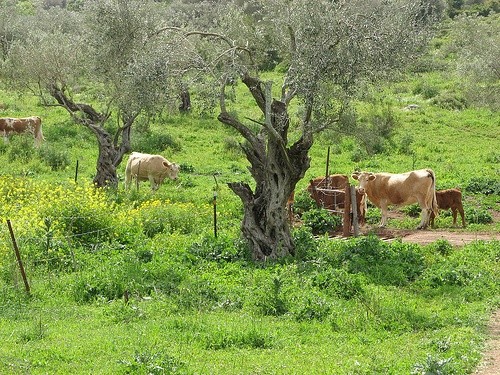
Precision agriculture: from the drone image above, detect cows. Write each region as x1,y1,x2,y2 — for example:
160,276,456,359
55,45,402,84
125,151,179,191
0,116,46,143
308,169,467,229
